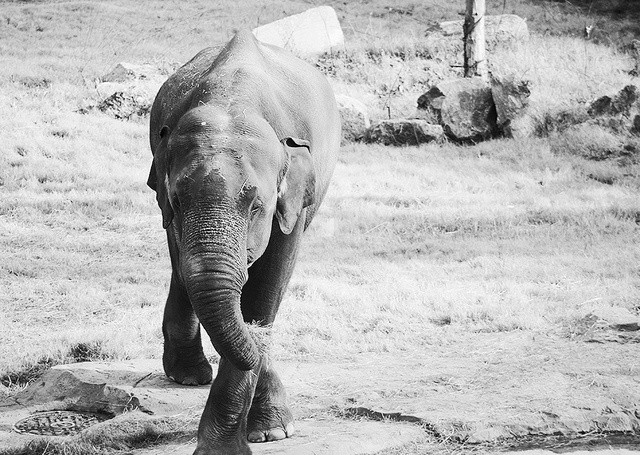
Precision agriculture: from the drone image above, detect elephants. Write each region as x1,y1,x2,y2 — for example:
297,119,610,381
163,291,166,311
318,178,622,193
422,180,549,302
147,28,345,454
